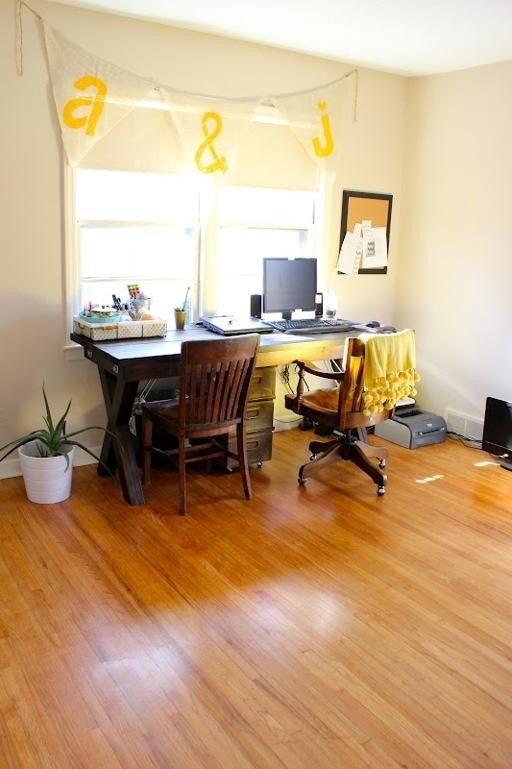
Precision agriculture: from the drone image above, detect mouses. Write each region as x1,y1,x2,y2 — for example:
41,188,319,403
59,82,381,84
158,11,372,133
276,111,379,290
365,321,380,328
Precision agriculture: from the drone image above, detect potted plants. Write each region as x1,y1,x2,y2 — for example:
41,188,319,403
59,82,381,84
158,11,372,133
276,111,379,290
174,286,191,329
0,379,117,505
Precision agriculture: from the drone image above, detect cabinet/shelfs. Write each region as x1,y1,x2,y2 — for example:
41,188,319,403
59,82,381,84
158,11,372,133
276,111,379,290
188,365,276,473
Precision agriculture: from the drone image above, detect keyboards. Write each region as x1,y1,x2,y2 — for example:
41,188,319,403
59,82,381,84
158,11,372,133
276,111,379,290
268,320,355,335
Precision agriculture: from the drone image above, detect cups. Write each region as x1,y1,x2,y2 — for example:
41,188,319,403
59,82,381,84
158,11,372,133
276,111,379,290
174,311,186,329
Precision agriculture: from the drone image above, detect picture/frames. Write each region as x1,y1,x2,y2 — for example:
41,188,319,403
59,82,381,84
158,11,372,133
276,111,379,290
337,190,392,274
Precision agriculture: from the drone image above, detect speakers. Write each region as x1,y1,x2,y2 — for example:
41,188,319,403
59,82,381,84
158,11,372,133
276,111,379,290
314,292,323,319
250,294,263,318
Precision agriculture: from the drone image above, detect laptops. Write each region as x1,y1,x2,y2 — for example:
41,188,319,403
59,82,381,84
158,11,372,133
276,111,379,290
202,315,274,335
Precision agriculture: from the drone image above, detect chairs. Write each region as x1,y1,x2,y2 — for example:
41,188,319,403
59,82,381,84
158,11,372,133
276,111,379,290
140,333,261,516
285,329,416,495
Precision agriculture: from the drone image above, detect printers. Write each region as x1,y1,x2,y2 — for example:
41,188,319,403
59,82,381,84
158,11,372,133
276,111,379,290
374,407,447,449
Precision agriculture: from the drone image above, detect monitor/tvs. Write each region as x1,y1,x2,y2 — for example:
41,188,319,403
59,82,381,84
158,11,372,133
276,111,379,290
481,397,512,471
263,257,317,320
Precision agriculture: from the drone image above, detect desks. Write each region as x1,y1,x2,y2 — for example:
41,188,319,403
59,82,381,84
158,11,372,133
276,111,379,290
70,319,398,506
